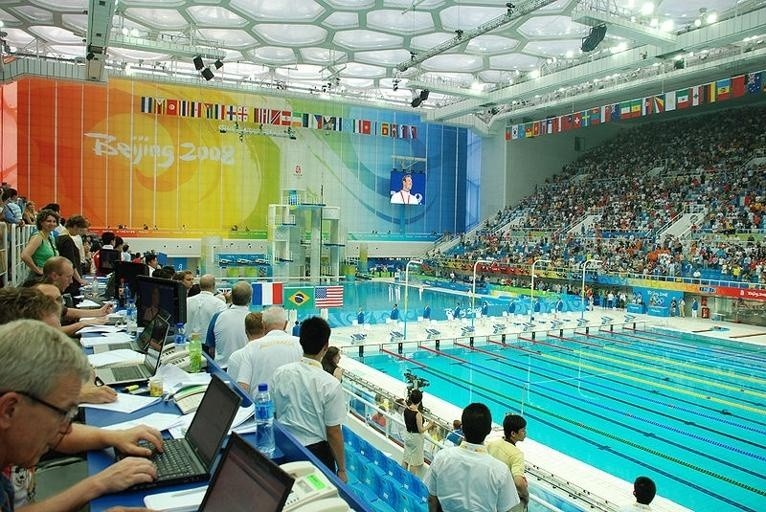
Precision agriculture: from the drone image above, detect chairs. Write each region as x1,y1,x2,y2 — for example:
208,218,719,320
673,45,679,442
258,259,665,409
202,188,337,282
424,182,766,299
336,423,429,512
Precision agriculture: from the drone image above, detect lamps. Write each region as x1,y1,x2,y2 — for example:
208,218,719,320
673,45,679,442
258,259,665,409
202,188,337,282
503,1,514,16
189,55,226,82
455,29,467,39
410,84,433,109
577,22,607,53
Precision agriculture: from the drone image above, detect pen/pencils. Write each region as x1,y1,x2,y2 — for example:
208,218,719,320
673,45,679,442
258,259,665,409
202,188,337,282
94,377,104,387
121,383,147,393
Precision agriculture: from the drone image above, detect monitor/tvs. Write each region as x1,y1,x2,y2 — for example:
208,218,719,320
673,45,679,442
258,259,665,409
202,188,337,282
99,250,120,272
137,274,187,329
114,260,148,297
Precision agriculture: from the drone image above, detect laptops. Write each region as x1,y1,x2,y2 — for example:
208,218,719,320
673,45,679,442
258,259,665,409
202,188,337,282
196,431,295,511
93,308,170,353
118,374,241,493
94,315,170,385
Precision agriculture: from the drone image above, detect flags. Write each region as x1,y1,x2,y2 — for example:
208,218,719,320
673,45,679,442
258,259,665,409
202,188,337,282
505,72,765,139
141,98,418,140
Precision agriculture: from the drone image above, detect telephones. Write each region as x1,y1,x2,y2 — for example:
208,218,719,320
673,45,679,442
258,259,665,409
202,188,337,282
161,342,207,372
278,460,357,512
174,381,230,415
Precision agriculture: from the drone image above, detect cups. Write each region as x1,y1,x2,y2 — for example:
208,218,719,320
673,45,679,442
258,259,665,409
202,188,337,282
150,379,165,397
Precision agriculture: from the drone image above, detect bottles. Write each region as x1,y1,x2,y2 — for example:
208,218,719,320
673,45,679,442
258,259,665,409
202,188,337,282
174,322,187,350
124,284,131,304
126,299,138,334
189,333,202,372
92,276,98,293
254,383,277,454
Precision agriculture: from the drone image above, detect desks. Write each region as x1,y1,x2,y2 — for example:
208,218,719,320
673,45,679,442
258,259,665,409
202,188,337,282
78,269,377,512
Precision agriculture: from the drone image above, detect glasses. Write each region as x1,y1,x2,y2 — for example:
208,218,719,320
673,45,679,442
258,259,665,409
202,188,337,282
15,390,78,422
